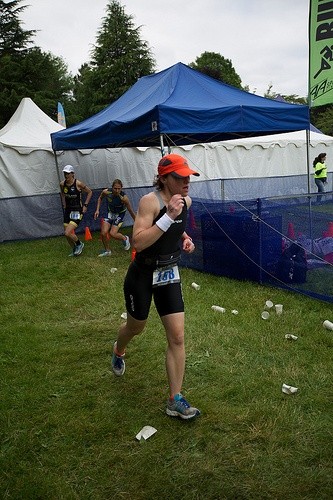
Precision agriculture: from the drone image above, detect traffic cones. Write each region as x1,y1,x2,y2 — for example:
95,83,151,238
187,207,197,231
100,217,110,241
85,226,92,241
285,222,296,241
71,228,75,235
281,238,285,254
131,247,137,262
322,220,333,264
229,204,235,213
299,231,307,257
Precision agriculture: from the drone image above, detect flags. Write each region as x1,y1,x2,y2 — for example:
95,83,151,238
309,0,333,107
58,102,66,127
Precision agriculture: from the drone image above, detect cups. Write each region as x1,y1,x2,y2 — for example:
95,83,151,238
136,425,157,440
211,305,225,313
264,300,273,310
323,320,333,330
282,383,299,394
191,282,200,290
275,304,284,314
111,268,118,273
261,311,270,320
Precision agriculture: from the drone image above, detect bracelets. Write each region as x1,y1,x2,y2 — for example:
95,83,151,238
156,213,175,231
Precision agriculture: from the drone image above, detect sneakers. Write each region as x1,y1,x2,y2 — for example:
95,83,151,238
111,341,125,377
123,235,130,251
98,249,112,257
73,241,84,256
166,393,200,419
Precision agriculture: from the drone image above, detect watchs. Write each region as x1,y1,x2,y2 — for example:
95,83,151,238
83,204,88,207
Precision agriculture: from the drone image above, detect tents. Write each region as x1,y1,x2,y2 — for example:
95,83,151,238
0,98,332,242
50,62,312,238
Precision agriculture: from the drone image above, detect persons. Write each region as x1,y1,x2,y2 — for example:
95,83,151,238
313,153,327,205
94,179,136,256
59,165,92,256
111,153,201,419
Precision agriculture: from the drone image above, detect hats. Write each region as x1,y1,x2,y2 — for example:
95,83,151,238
63,164,74,174
158,154,200,179
